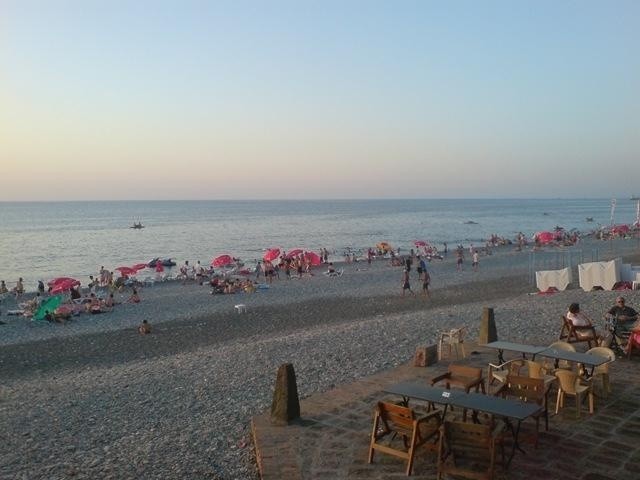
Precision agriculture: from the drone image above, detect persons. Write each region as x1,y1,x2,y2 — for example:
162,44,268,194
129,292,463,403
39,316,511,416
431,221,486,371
138,319,152,335
517,224,640,252
2,267,139,321
155,258,172,281
565,303,614,348
368,233,510,272
402,255,430,297
181,248,357,294
620,311,640,355
604,295,640,346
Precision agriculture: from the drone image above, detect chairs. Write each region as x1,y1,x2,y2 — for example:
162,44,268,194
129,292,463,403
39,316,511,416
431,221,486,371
433,417,507,479
558,313,639,360
578,347,616,394
500,375,550,448
485,358,521,389
439,325,468,361
429,363,482,420
528,358,556,409
554,369,594,417
368,398,440,477
367,313,640,479
545,342,576,370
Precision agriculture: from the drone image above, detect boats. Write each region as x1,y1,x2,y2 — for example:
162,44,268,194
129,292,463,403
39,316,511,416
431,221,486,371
131,220,146,229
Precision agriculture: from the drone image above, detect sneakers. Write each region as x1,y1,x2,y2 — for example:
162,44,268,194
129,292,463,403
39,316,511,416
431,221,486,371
619,345,629,355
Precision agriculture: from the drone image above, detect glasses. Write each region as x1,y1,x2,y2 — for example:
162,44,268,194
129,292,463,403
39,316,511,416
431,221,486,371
617,300,624,303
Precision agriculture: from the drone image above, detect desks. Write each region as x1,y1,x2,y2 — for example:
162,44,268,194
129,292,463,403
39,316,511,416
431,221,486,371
481,340,546,373
539,348,611,404
451,393,541,466
380,381,465,451
